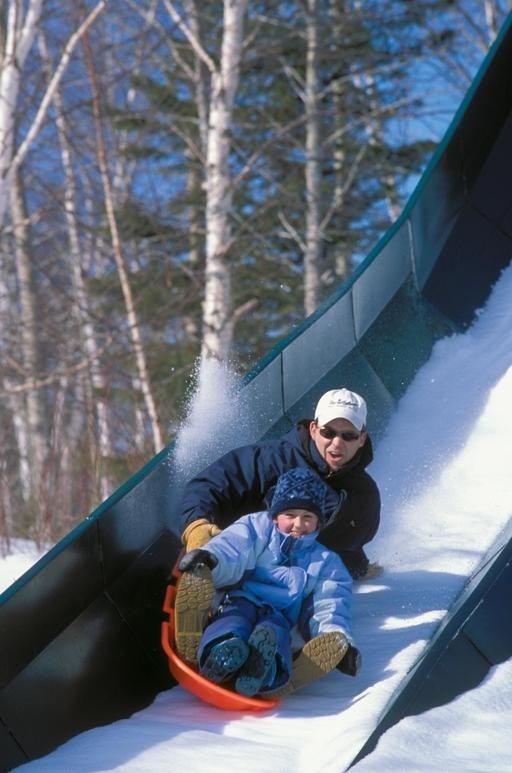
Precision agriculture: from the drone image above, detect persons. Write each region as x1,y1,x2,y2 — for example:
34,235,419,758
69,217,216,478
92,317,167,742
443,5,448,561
179,385,382,586
180,465,363,697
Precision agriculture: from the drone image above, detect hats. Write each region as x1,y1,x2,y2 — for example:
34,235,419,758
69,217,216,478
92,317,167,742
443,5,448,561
314,388,368,431
270,467,325,521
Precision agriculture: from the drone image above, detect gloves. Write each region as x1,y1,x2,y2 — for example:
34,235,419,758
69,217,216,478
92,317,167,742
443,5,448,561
335,643,360,677
180,518,222,554
178,549,211,572
355,560,385,580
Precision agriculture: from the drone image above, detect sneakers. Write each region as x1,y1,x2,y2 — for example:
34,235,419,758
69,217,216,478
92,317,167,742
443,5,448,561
199,637,249,685
174,562,213,668
235,623,278,697
260,631,349,701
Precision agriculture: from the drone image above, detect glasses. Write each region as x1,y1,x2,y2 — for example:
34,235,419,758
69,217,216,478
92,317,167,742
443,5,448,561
316,421,362,441
